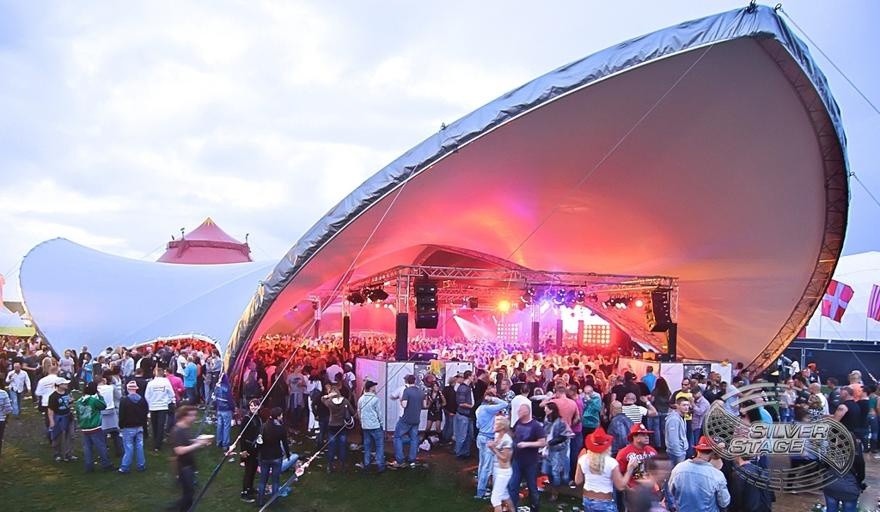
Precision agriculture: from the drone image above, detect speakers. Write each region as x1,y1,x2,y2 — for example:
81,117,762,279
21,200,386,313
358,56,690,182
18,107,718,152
415,283,438,328
668,323,677,358
395,313,407,358
645,291,671,330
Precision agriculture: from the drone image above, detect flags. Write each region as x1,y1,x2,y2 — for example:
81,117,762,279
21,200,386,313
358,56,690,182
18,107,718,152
821,279,854,323
866,283,880,322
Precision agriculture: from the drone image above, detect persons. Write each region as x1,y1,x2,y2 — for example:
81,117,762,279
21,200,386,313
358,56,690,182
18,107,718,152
0,329,880,508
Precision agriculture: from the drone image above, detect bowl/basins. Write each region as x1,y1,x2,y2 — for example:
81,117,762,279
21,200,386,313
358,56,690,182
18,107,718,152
198,438,215,446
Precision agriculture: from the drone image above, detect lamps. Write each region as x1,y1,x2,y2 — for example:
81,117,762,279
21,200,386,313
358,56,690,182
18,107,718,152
520,278,587,305
601,290,633,309
347,281,389,304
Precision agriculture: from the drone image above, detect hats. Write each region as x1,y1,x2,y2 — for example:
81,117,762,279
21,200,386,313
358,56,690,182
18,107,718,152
585,427,615,452
53,375,72,385
627,422,653,441
692,435,718,451
364,380,378,388
